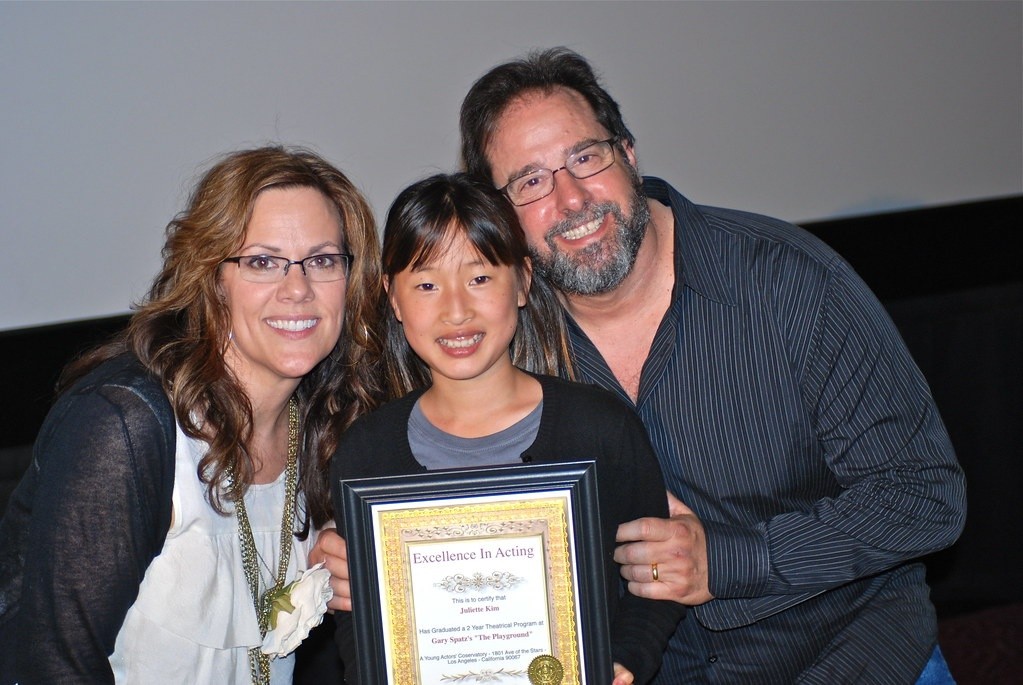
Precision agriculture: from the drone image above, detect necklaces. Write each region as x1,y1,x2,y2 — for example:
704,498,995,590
223,391,301,685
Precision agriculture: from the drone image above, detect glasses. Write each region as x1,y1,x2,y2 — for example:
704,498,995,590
497,134,621,205
220,254,354,282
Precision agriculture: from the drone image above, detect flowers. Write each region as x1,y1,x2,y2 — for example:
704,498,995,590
260,557,335,661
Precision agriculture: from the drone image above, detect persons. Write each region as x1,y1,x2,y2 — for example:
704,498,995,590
460,44,967,685
292,172,678,685
0,145,383,685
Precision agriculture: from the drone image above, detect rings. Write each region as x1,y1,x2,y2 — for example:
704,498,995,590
652,563,658,580
